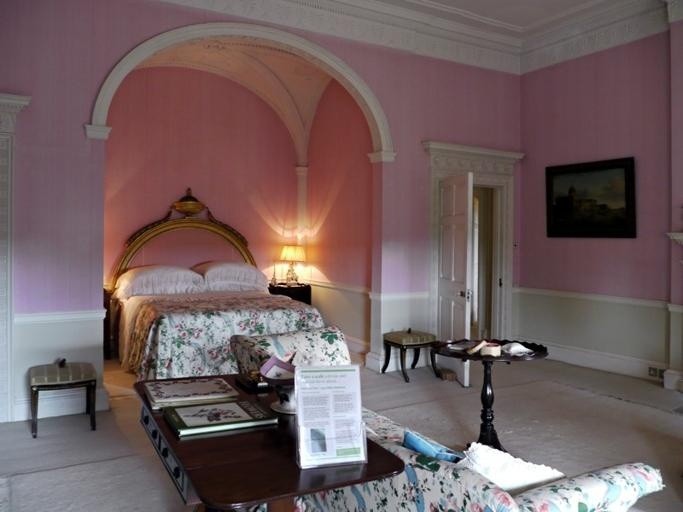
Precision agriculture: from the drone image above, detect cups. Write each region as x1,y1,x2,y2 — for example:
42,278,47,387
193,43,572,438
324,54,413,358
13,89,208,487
480,344,501,357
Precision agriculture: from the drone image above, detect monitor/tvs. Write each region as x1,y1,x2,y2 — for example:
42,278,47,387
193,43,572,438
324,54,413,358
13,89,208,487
545,157,636,238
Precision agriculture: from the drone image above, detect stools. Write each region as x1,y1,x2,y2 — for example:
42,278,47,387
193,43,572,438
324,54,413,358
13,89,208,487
28,362,97,438
382,331,440,383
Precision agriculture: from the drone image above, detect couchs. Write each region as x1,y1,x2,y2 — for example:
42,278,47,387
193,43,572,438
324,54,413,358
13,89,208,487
231,323,666,512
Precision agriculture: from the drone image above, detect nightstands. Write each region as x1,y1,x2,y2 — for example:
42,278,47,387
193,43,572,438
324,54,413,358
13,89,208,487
104,287,113,359
268,282,311,307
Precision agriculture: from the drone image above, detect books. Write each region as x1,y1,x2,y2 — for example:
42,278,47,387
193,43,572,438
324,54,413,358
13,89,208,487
142,376,279,440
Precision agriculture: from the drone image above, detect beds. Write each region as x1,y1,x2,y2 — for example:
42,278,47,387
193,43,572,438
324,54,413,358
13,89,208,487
110,187,317,380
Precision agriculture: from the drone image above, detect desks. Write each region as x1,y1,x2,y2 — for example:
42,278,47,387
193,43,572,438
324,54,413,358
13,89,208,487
432,339,548,453
133,374,405,512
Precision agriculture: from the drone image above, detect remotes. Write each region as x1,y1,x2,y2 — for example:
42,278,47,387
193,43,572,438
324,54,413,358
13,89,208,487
445,339,466,345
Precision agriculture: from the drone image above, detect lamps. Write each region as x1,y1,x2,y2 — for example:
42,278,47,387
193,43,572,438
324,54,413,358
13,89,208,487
280,245,306,286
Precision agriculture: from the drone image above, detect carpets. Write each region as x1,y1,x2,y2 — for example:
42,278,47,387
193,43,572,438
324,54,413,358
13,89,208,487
0,379,683,512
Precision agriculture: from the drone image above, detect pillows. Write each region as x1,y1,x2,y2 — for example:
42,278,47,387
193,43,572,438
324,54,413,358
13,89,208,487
117,285,203,299
458,442,568,497
115,264,203,294
191,261,267,284
402,427,464,463
204,281,268,291
